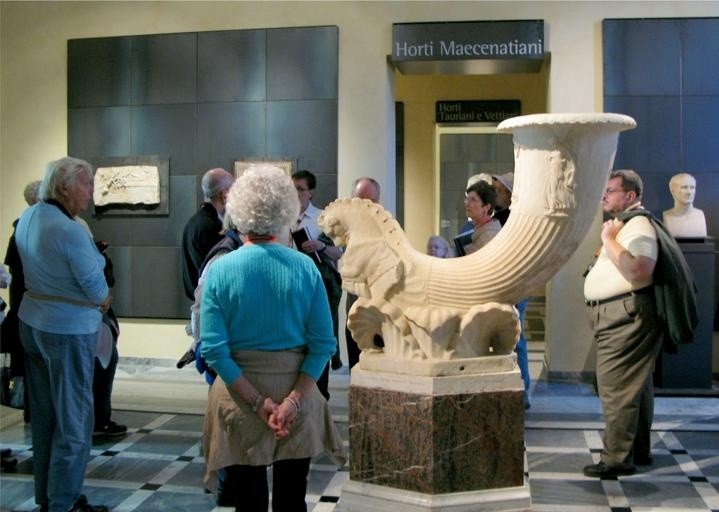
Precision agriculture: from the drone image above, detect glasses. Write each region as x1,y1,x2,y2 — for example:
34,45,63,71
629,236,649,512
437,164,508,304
606,188,630,193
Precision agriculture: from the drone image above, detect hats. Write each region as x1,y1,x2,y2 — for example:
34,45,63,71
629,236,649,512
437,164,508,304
491,172,514,194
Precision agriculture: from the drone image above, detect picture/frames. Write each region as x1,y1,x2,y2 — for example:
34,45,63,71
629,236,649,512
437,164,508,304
230,154,298,185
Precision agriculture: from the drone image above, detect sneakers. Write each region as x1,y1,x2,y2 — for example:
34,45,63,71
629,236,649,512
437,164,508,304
92,421,128,437
0,449,18,471
68,494,109,512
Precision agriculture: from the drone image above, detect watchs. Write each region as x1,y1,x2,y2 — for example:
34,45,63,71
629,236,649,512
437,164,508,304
321,241,327,253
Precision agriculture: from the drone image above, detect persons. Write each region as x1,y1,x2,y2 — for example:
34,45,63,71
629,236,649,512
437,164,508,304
181,167,234,299
92,239,127,436
5,179,42,423
198,162,349,512
662,172,706,239
345,177,381,371
15,157,114,511
287,170,347,400
288,228,294,250
457,172,497,235
426,235,450,260
491,172,532,410
464,179,503,253
582,168,665,477
189,181,248,508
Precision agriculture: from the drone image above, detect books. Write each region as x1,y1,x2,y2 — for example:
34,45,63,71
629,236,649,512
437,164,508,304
292,225,321,266
452,229,475,257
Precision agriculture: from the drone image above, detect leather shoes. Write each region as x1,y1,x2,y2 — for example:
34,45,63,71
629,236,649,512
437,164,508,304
582,460,637,479
632,452,652,465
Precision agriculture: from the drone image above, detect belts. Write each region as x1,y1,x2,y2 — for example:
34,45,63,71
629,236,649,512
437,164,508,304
585,284,654,307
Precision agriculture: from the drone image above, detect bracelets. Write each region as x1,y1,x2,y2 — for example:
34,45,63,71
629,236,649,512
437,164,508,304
251,394,266,413
284,398,299,414
290,391,302,410
